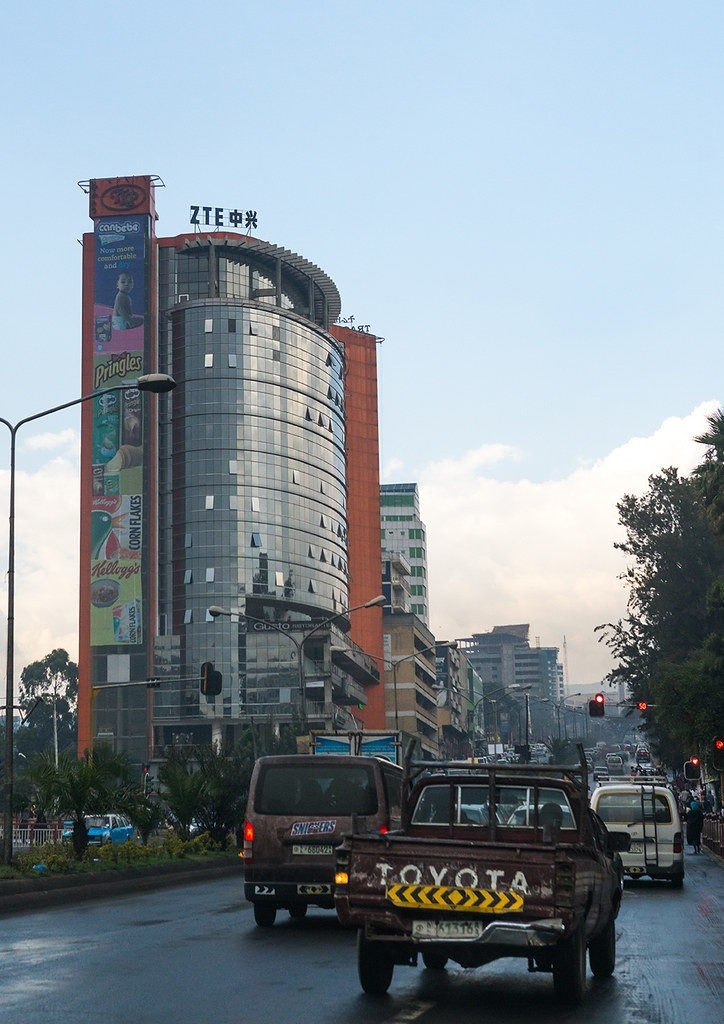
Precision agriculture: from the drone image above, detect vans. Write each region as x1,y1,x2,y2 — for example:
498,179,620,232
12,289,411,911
243,753,410,926
585,776,686,884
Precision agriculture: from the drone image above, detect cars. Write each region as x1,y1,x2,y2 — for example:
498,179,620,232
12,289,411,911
78,814,138,847
476,734,665,779
453,804,576,831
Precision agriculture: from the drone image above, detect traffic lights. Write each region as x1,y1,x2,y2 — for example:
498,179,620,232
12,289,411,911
712,735,724,771
684,756,702,781
636,701,647,711
588,693,605,717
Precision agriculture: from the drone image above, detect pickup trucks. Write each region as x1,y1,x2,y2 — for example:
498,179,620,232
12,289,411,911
333,738,631,1004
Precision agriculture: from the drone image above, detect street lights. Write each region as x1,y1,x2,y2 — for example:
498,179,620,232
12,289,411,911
0,372,176,868
329,639,458,764
431,683,585,769
208,595,388,736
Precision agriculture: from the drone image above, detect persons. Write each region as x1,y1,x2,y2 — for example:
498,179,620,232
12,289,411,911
631,760,716,821
536,802,564,831
110,271,143,330
297,780,324,813
685,802,704,854
